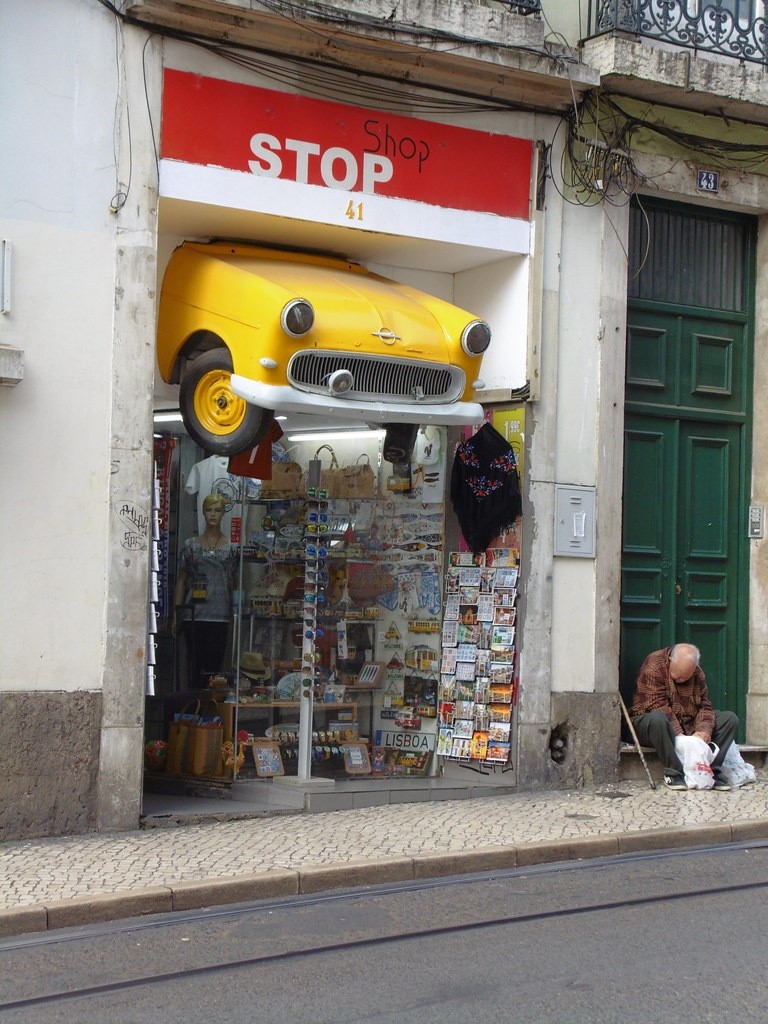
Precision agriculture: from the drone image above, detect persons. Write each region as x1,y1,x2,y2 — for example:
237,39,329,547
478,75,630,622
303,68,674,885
625,643,739,791
171,494,239,689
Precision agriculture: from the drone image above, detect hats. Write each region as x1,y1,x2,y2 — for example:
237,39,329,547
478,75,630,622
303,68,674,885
231,652,271,680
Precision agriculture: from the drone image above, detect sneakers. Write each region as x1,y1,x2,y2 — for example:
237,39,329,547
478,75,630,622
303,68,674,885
663,774,688,790
712,777,732,790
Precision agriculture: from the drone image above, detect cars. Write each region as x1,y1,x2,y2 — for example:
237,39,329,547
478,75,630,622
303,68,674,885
160,237,491,463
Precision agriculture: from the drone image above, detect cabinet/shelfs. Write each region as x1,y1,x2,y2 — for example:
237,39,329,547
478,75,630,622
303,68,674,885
229,494,388,622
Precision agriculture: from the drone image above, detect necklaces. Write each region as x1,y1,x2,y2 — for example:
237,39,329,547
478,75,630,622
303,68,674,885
204,532,222,554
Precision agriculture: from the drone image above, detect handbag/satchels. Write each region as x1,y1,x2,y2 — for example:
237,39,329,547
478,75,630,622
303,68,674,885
165,700,224,778
258,462,307,501
718,740,757,790
343,454,373,499
675,736,719,789
299,444,345,498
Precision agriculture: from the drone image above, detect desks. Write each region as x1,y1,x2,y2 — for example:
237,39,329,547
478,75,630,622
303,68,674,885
214,700,357,772
330,686,383,764
